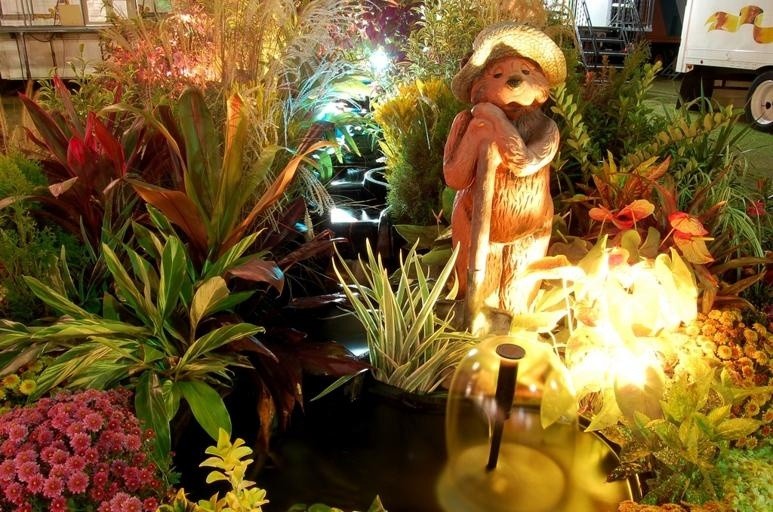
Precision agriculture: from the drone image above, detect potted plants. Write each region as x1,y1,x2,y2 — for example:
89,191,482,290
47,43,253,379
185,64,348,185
316,230,482,474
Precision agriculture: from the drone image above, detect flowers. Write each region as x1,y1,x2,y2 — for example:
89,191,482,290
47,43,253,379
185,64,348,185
1,384,179,512
